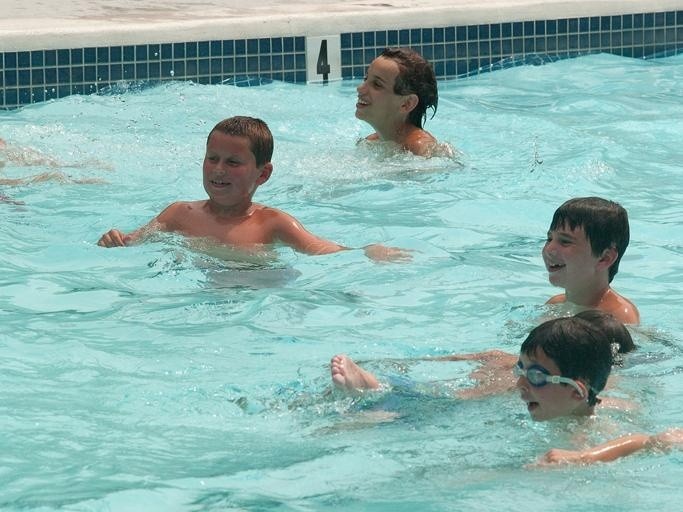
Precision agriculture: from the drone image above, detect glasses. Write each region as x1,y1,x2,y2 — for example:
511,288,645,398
510,361,585,398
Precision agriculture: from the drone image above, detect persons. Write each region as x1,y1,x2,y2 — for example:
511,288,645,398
515,314,652,470
327,194,640,415
89,115,418,289
352,46,466,171
